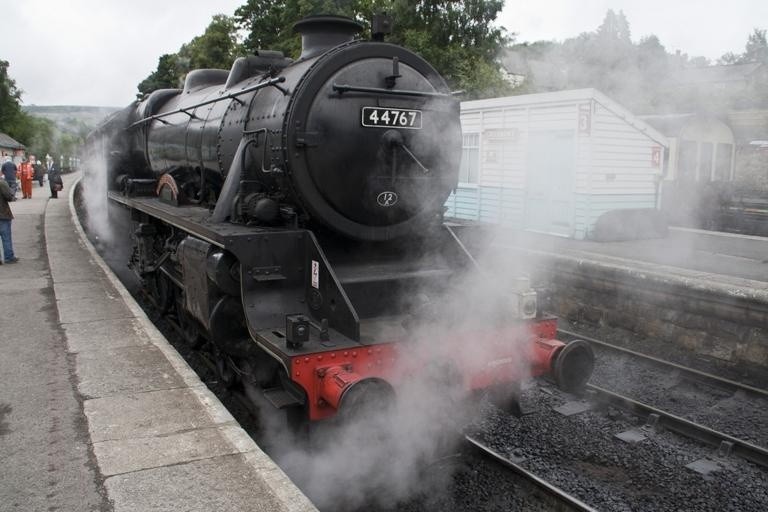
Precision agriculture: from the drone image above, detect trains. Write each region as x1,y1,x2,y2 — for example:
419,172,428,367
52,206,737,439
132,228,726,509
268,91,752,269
81,12,595,454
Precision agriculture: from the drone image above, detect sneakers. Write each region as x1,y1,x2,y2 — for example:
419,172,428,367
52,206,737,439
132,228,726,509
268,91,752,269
6,257,19,264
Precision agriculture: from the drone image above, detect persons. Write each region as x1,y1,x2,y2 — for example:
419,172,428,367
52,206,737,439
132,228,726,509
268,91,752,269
0,153,59,199
0,172,18,264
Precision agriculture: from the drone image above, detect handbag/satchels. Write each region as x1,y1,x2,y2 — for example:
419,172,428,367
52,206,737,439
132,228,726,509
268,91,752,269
54,184,61,191
